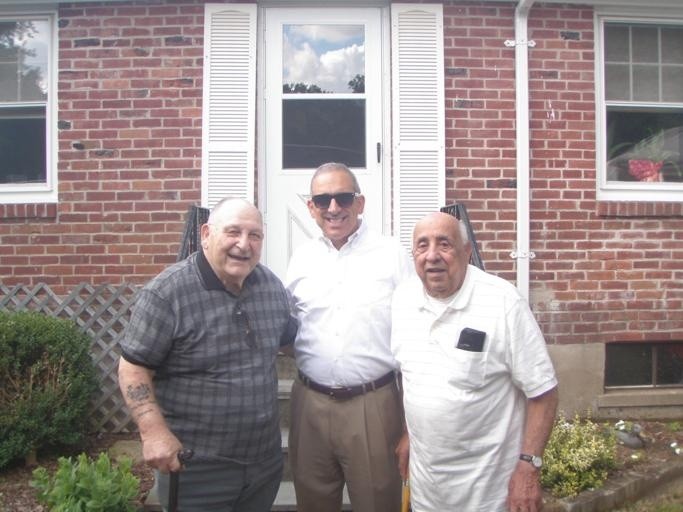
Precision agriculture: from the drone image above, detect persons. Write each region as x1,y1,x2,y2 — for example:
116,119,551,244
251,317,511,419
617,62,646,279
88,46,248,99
117,195,298,512
274,161,412,512
390,211,560,511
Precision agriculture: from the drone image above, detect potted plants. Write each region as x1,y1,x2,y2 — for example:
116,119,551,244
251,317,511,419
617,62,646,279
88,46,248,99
607,129,682,182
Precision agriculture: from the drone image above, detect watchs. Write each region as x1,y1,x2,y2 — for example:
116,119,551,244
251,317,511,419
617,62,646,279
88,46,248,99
518,453,544,470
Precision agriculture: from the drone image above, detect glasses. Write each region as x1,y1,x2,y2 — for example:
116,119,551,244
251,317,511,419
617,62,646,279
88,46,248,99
232,300,258,349
310,192,358,208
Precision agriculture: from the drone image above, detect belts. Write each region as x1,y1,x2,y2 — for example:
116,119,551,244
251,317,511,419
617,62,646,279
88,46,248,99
296,368,394,400
